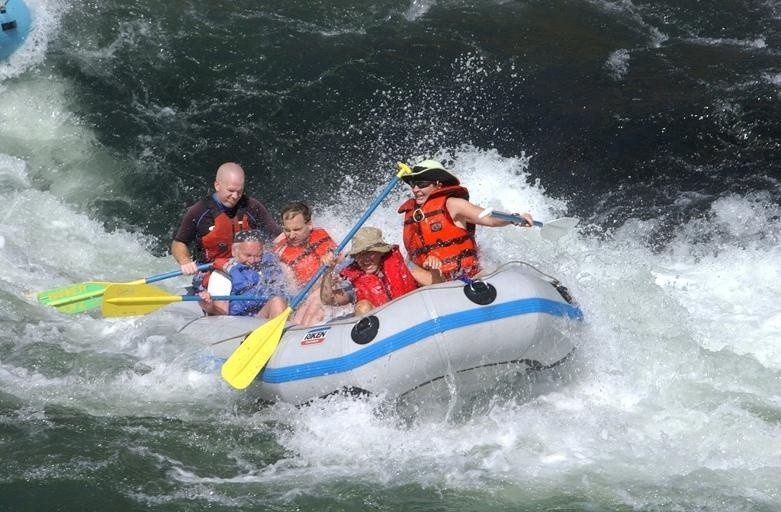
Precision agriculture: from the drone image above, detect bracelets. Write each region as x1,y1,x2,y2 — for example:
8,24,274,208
510,212,520,225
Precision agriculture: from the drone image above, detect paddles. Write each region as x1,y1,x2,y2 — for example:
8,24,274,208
221,160,411,388
100,281,297,319
36,262,214,315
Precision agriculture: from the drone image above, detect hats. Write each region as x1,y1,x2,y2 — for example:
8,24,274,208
349,227,393,255
401,160,460,186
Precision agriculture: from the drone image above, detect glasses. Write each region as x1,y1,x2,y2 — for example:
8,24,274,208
409,181,437,188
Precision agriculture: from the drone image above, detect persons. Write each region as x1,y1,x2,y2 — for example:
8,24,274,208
319,226,444,318
195,229,329,328
397,156,537,281
169,161,285,317
269,201,347,299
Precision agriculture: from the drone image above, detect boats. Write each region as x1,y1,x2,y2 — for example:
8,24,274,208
131,269,594,420
